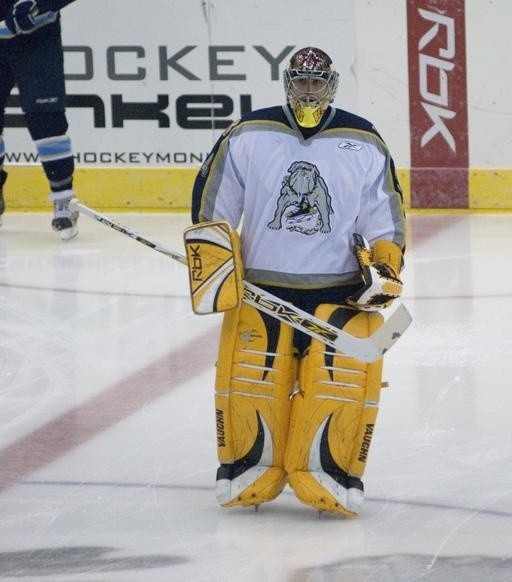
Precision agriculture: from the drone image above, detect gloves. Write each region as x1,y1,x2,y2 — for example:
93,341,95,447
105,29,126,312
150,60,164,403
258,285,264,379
345,231,405,314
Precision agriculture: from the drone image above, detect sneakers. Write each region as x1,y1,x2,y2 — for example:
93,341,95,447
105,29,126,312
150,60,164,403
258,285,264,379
49,189,79,232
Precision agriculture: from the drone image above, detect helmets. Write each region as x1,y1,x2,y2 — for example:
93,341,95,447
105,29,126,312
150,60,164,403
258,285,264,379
282,46,340,129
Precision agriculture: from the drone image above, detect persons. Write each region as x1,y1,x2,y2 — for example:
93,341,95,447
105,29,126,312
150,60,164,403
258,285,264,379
0,0,80,232
183,46,406,518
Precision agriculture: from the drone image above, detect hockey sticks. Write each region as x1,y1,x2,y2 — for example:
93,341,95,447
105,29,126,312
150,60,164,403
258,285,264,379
67,197,412,364
